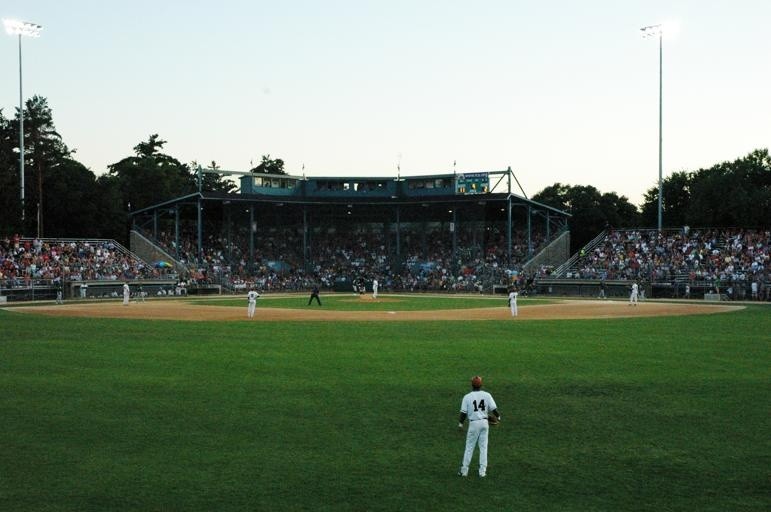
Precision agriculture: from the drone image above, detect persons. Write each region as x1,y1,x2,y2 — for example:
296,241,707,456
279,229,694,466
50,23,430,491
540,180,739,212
507,287,519,317
245,287,261,318
455,374,502,479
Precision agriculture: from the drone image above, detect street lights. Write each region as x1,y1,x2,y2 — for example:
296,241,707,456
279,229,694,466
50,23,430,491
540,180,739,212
2,18,42,216
641,24,669,231
